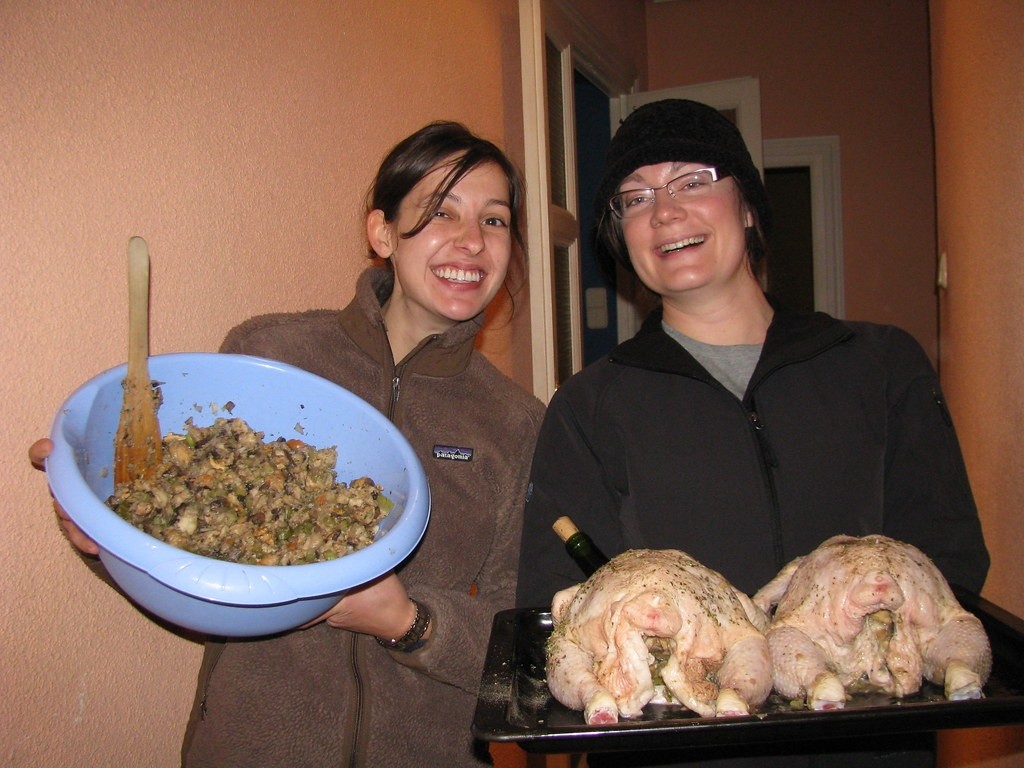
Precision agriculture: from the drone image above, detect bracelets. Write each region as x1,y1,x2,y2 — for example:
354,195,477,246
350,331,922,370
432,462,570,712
377,600,430,653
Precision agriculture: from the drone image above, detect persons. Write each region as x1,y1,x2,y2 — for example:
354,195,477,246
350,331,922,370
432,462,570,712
518,97,990,767
28,120,551,768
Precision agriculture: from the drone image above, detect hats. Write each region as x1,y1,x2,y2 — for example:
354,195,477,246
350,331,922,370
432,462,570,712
600,97,773,260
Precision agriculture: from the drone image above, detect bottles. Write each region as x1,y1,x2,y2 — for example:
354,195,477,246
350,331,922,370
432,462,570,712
564,531,608,579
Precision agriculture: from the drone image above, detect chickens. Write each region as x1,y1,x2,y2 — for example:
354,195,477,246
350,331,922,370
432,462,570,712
544,533,992,725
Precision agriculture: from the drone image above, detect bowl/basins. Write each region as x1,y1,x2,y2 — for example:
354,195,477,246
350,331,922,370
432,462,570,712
45,351,433,641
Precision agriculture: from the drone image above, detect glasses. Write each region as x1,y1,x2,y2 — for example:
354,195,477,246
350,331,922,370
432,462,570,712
607,166,731,219
